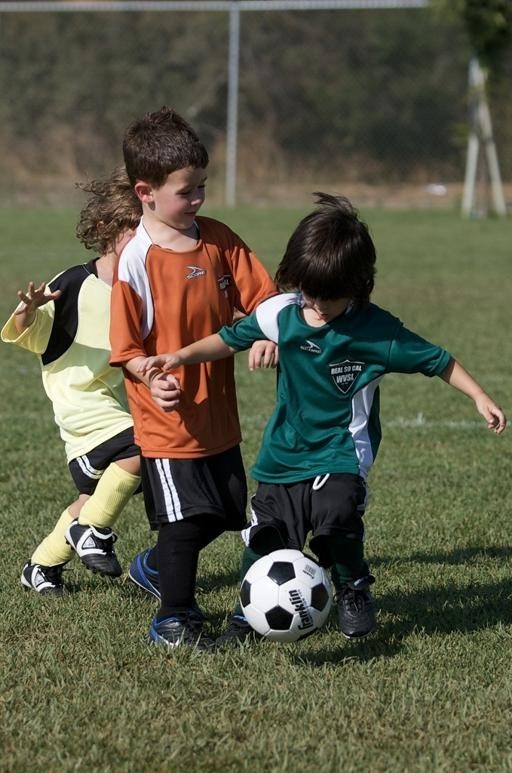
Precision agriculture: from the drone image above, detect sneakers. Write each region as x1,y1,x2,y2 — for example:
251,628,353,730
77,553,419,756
150,610,249,654
21,517,160,599
336,575,375,640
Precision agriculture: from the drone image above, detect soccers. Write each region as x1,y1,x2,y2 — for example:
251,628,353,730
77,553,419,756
239,548,331,642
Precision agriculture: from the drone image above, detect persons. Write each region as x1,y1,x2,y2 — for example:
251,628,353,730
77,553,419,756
137,190,506,649
0,162,141,597
110,104,279,651
